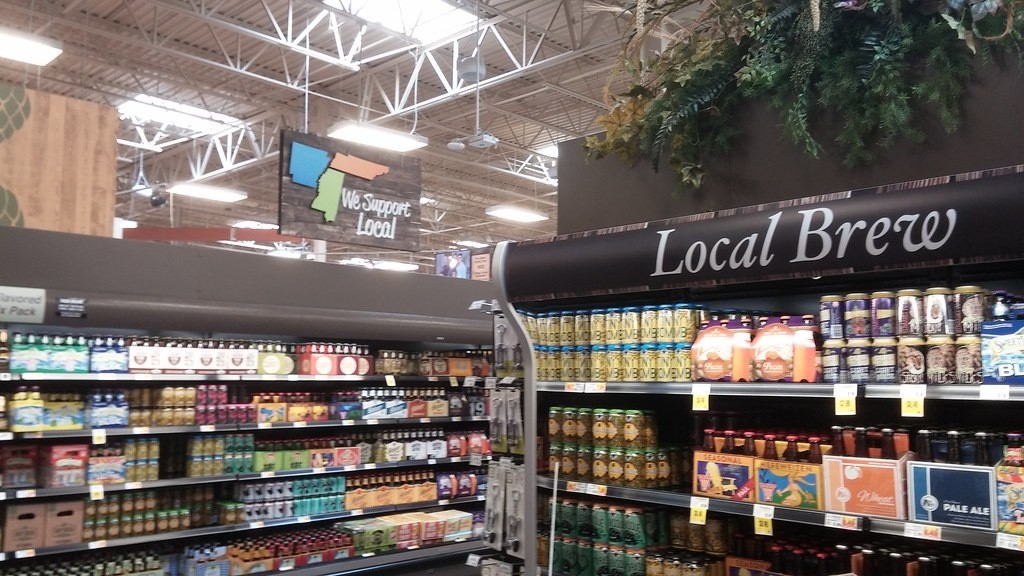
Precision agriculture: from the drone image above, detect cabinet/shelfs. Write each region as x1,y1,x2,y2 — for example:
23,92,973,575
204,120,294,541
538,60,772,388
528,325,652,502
491,163,1024,576
0,373,497,576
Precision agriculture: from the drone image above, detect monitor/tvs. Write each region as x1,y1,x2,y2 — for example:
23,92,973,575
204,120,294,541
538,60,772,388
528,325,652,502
434,248,471,281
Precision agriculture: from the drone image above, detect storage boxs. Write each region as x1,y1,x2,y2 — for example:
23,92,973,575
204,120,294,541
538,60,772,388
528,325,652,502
692,448,1024,536
0,345,490,576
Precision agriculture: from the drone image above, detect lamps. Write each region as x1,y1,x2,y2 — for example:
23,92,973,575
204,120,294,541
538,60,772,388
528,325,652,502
485,154,551,225
327,24,429,155
0,0,64,66
309,0,486,46
165,143,247,203
114,71,246,139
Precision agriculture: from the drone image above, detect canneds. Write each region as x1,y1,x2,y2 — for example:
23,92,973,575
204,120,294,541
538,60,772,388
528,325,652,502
546,498,1024,576
821,286,1024,383
122,437,160,482
546,407,741,488
516,304,776,386
124,384,257,428
84,474,345,542
186,434,255,478
